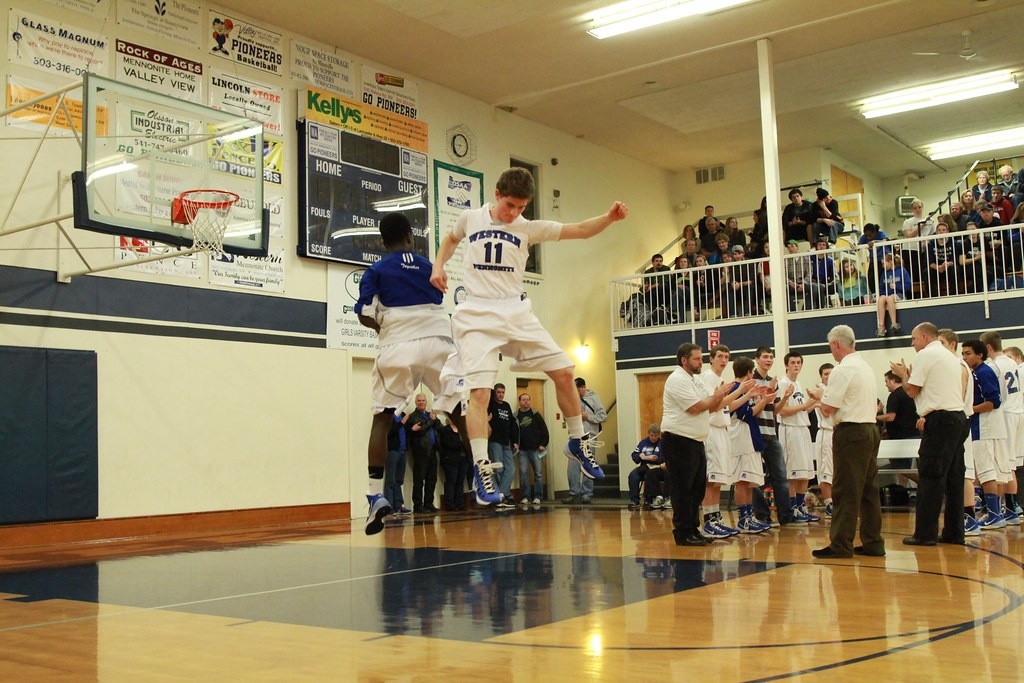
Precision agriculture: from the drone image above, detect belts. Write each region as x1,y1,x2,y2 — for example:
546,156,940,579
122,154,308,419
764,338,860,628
933,410,951,413
839,422,855,425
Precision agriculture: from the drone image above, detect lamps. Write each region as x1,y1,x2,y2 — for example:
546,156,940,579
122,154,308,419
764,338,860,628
585,0,759,41
857,73,1019,120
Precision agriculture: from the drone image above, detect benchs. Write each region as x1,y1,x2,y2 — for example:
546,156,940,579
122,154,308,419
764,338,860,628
875,439,924,474
643,477,708,501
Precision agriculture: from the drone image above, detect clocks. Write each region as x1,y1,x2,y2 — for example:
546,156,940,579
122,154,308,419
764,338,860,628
451,134,468,158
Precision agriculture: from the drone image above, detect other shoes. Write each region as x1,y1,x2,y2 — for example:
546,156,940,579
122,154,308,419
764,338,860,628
876,329,886,338
890,324,900,333
391,496,673,515
938,535,950,543
812,546,852,558
854,547,870,555
902,536,937,546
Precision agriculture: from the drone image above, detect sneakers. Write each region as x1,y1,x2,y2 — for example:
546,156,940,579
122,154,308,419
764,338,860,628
365,493,393,535
472,459,505,506
392,505,412,515
672,502,833,546
964,508,1024,536
563,430,606,481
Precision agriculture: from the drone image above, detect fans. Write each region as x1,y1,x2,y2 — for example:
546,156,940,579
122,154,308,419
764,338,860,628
911,26,1009,63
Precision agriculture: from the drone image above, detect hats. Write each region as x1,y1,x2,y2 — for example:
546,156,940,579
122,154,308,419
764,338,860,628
785,239,798,247
574,377,585,386
816,188,828,200
731,245,744,254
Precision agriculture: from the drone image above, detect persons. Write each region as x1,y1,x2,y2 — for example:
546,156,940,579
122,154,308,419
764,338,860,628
890,321,969,546
877,370,918,491
835,252,912,336
384,393,472,517
814,362,835,518
856,223,896,302
354,212,472,535
627,423,673,509
639,187,845,326
752,344,808,528
429,167,629,505
777,351,821,523
807,325,887,559
726,355,777,534
487,383,549,508
901,198,936,282
924,164,1024,300
560,377,607,504
938,329,1024,537
660,343,736,546
700,344,768,539
